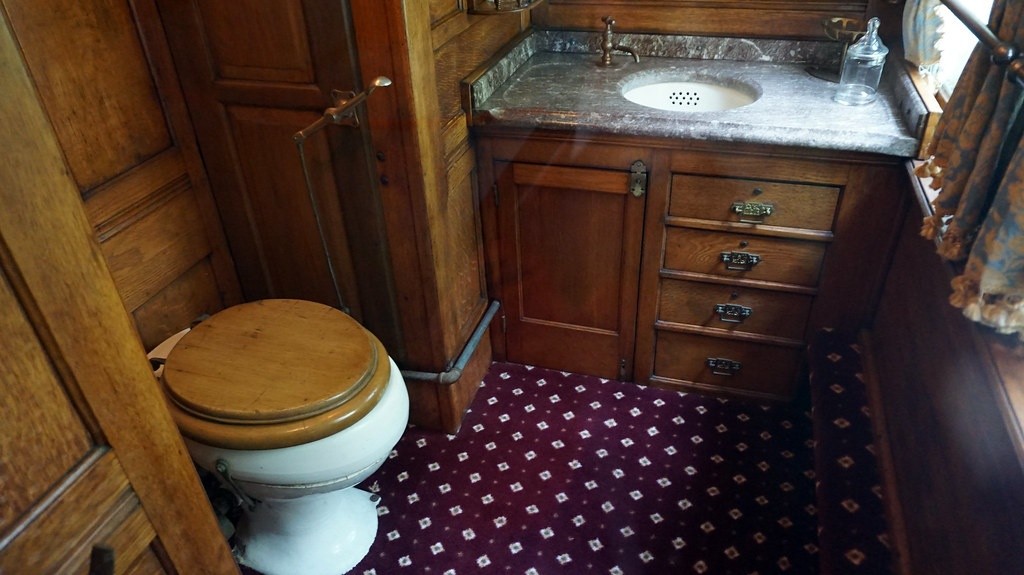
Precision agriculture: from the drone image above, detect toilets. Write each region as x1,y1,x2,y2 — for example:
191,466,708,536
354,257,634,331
141,290,410,575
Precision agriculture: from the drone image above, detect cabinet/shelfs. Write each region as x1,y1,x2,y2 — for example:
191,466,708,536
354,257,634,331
469,130,899,406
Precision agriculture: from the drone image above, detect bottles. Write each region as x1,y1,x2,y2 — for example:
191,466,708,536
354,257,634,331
834,16,890,107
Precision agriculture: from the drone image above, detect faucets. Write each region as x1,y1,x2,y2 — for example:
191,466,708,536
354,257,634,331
595,14,640,67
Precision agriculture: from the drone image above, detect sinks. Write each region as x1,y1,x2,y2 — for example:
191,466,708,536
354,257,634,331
616,66,764,113
